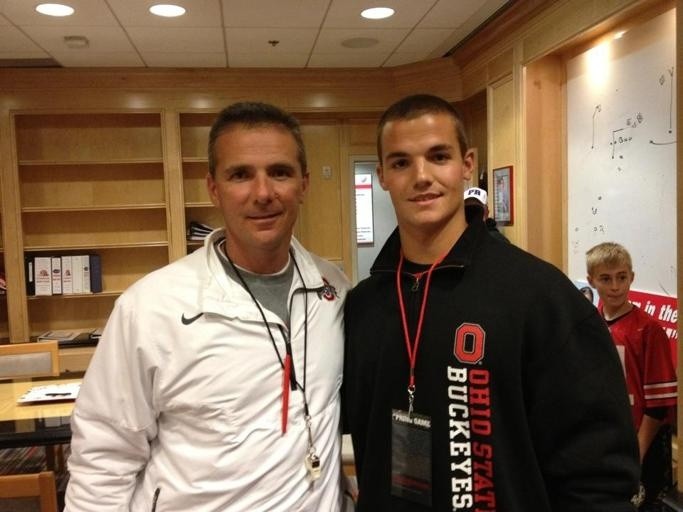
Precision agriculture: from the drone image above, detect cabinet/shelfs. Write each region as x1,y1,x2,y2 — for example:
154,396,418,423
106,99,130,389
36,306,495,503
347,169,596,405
0,97,223,371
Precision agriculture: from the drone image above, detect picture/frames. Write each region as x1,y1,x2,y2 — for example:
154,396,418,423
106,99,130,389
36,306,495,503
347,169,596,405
491,166,512,227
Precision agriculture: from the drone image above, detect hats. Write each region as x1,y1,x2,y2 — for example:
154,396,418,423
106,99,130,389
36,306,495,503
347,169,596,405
464,187,487,204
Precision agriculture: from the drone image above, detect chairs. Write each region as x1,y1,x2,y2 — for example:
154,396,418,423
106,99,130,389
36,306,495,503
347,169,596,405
0,339,63,512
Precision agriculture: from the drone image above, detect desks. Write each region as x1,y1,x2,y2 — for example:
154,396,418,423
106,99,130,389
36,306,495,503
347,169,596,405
0,371,83,452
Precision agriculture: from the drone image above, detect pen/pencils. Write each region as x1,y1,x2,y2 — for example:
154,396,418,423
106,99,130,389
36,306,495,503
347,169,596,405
282,343,292,434
46,393,71,397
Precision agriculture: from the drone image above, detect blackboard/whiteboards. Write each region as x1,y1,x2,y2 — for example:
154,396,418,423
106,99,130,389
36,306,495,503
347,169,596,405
560,10,683,299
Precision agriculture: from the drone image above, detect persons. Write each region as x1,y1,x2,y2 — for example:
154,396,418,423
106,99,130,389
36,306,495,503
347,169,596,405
58,100,357,512
585,241,680,511
335,93,643,511
